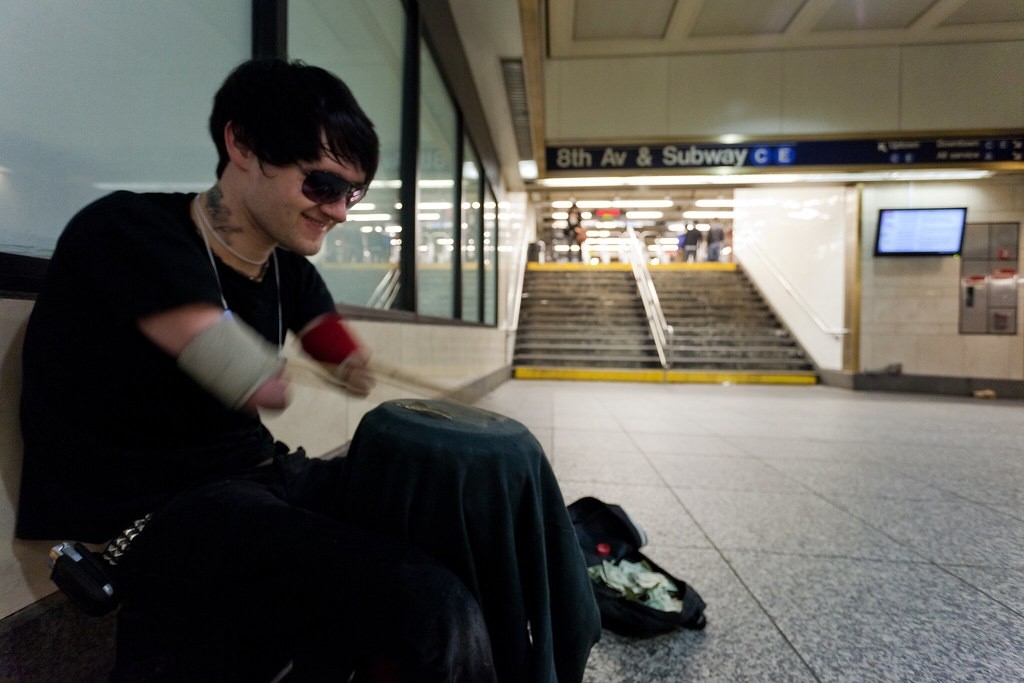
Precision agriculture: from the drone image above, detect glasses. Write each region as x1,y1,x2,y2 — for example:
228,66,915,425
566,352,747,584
292,162,369,209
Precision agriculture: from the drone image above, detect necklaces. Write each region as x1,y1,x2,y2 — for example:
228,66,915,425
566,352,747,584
195,195,283,360
198,192,268,265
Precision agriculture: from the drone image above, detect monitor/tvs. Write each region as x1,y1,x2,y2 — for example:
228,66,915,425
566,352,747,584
874,207,967,257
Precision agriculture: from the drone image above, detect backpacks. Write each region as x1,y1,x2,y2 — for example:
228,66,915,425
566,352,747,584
567,497,707,640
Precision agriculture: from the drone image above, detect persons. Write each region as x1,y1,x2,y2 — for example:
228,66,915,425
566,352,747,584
707,218,723,262
19,57,602,683
676,220,703,261
568,203,584,263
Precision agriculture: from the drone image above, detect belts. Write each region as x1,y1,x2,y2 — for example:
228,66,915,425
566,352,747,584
103,506,152,567
